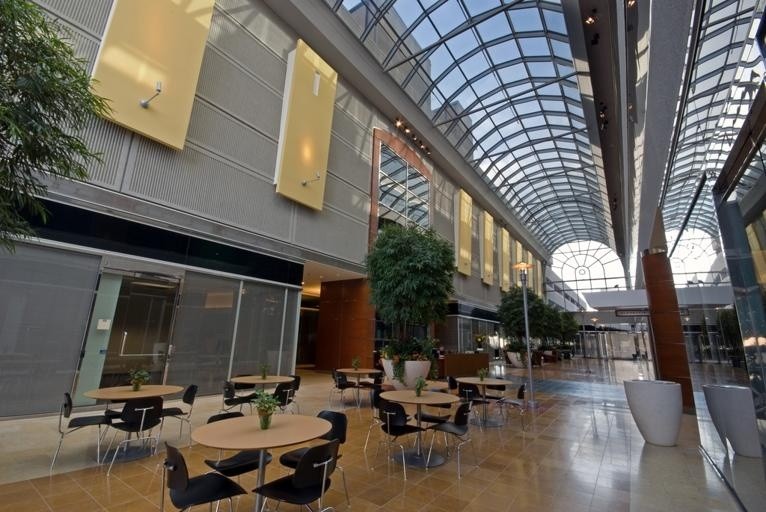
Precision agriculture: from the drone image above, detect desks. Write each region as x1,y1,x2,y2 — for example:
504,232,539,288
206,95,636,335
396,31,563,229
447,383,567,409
190,412,333,511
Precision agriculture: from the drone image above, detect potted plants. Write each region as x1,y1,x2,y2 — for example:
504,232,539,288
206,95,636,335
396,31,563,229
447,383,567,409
476,367,489,381
251,385,282,431
414,376,428,397
352,359,362,371
260,365,269,379
363,220,457,392
494,282,580,368
127,367,151,391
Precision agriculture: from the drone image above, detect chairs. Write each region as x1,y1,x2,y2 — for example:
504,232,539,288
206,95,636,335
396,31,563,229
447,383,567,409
50,384,197,476
218,372,302,415
162,439,249,511
279,410,353,511
447,374,528,434
251,438,341,511
203,412,273,511
330,364,385,409
363,391,473,480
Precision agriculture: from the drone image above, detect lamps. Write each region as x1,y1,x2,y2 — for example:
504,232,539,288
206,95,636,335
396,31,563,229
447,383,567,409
300,170,322,187
139,81,162,108
585,7,601,24
394,117,433,158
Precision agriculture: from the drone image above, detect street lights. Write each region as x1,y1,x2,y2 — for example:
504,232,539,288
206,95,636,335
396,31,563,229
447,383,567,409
590,316,603,363
578,306,592,374
512,261,542,409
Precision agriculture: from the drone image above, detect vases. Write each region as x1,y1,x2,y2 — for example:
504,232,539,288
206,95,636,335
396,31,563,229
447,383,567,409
701,383,762,459
622,380,682,447
